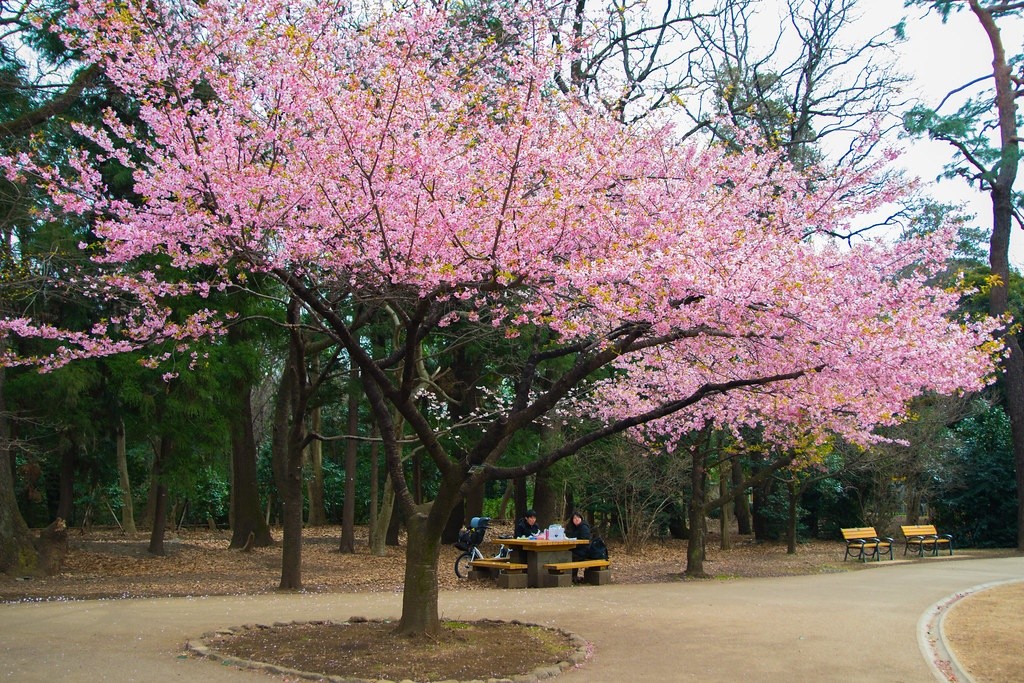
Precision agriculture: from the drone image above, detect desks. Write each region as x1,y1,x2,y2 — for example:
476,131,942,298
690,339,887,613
492,538,590,588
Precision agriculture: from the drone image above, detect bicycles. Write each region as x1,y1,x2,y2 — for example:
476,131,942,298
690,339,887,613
454,516,514,579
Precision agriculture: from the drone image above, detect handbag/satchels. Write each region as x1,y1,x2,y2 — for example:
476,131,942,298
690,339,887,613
588,537,609,561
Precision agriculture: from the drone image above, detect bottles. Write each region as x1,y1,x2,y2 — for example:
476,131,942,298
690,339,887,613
546,529,549,540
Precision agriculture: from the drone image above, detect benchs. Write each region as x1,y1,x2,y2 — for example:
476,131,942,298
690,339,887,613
840,526,894,562
468,561,527,589
900,524,953,557
476,558,509,561
544,559,610,587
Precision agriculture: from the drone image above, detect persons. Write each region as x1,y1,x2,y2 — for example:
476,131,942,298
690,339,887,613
565,512,591,582
514,509,539,561
